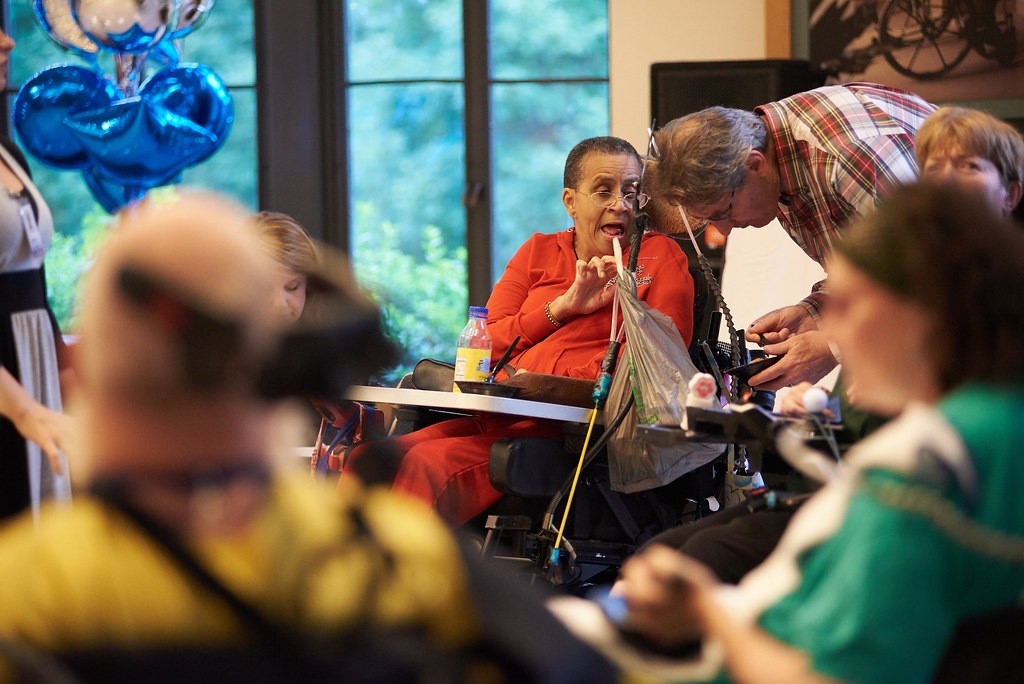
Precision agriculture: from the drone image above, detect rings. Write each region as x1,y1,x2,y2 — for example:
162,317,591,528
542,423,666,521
789,383,793,387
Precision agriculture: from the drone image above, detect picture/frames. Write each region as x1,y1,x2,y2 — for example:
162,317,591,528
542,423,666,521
790,0,1024,121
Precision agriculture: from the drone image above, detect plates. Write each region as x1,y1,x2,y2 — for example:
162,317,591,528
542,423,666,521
453,381,527,399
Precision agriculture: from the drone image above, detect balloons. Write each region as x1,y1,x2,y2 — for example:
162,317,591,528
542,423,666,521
13,1,235,214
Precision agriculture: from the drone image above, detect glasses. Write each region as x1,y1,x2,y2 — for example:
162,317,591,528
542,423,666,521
575,190,651,209
686,191,735,221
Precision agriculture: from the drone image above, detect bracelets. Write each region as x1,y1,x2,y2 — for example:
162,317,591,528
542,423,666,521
544,300,563,330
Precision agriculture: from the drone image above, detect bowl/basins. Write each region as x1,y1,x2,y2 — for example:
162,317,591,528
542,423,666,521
724,353,783,385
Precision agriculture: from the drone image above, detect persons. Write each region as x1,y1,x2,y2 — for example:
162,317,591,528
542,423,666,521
0,136,694,684
0,24,89,536
546,81,1023,684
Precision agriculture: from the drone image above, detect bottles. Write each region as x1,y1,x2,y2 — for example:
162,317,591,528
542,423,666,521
453,308,491,393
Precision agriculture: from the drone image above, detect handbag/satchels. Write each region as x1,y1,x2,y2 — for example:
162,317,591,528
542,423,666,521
412,358,455,392
603,270,729,493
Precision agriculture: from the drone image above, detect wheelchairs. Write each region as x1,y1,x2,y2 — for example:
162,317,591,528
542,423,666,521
309,264,751,593
